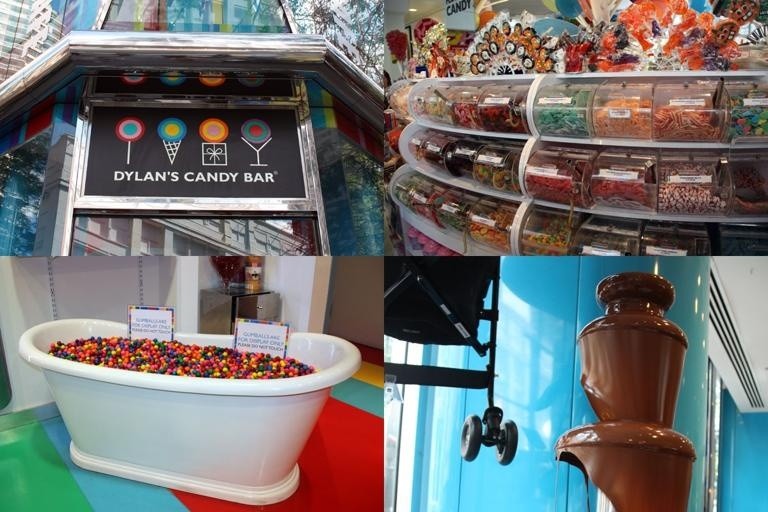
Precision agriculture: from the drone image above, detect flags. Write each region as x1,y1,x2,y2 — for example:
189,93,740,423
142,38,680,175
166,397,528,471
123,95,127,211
47,334,316,379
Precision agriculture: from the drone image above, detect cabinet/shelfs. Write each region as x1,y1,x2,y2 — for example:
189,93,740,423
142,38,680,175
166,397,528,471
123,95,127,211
513,68,767,256
200,285,281,336
390,71,544,257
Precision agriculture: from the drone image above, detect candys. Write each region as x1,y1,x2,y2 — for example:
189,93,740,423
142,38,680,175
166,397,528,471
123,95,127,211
411,0,768,76
157,117,187,165
47,335,316,379
395,83,768,255
199,118,229,143
240,118,273,167
115,116,146,166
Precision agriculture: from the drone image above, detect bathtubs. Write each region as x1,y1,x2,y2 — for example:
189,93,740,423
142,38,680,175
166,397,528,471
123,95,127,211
17,317,366,507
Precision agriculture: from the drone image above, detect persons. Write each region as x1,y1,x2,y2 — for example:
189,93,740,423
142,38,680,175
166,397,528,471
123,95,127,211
733,0,765,44
384,70,393,110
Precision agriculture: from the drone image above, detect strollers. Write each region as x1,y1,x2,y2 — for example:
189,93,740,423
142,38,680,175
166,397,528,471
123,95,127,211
382,256,520,468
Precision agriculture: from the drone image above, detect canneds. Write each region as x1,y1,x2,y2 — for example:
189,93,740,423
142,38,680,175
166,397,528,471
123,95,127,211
122,72,263,86
